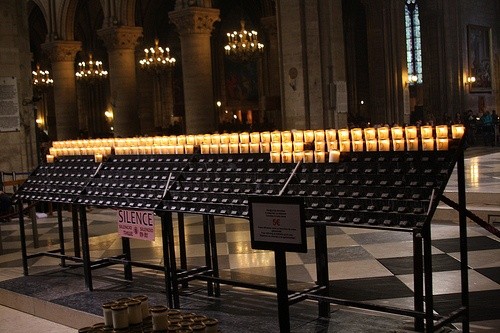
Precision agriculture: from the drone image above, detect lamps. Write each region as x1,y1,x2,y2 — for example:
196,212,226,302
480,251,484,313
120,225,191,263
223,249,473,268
224,19,265,60
31,65,55,90
75,54,109,84
139,38,176,75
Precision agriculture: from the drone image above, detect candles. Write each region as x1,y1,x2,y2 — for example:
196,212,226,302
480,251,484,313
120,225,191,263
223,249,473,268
79,300,219,333
47,125,465,164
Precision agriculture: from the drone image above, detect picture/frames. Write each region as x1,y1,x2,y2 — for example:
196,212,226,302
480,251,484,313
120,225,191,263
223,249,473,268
465,21,494,94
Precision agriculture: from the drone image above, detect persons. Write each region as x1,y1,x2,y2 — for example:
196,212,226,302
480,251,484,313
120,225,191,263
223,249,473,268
131,116,281,137
348,108,500,148
36,122,125,214
0,192,14,222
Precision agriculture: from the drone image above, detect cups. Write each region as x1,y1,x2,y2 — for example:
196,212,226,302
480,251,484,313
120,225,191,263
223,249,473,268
393,139,404,151
315,150,325,163
405,126,417,139
377,127,389,140
452,124,464,139
200,128,377,153
436,124,448,138
422,139,434,151
303,150,313,163
328,150,340,162
293,150,303,163
270,151,280,163
407,138,418,151
436,138,449,150
421,126,433,139
46,134,195,163
391,127,403,140
378,139,390,151
281,150,292,163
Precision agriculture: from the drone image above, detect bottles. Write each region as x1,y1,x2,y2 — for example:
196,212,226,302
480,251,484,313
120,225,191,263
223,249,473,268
78,295,218,333
